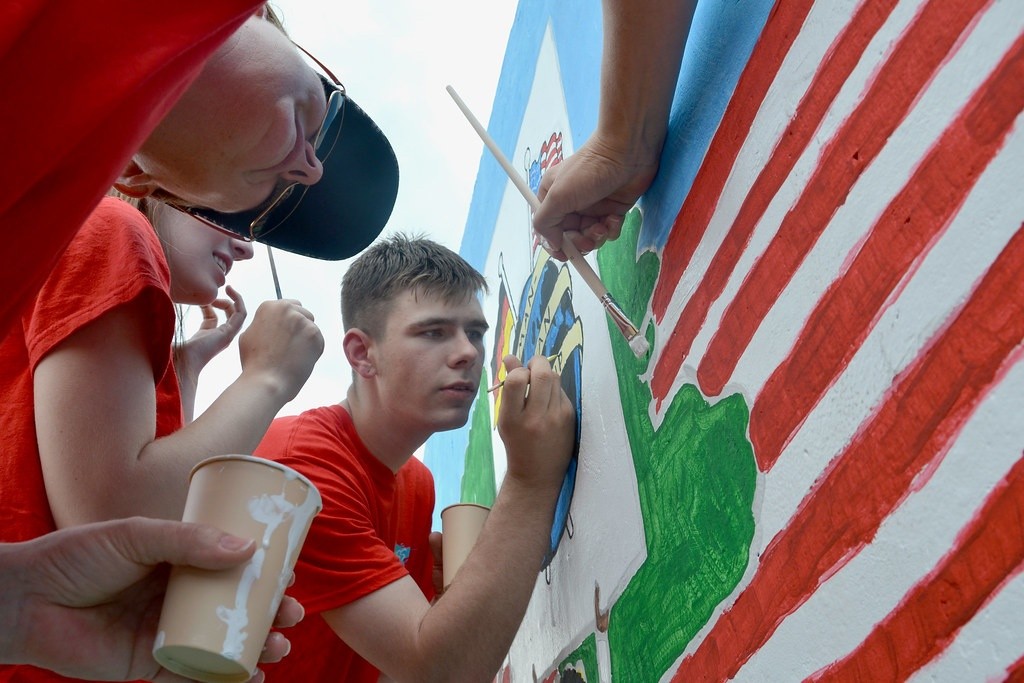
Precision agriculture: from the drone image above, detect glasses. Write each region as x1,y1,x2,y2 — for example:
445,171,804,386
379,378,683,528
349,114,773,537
173,41,346,242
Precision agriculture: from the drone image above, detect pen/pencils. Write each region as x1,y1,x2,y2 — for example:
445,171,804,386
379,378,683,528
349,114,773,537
487,355,558,393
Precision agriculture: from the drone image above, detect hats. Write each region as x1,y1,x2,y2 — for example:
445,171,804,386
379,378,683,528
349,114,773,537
186,70,400,262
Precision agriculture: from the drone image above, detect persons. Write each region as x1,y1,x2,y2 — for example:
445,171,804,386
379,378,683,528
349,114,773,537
0,0,698,683
252,230,577,683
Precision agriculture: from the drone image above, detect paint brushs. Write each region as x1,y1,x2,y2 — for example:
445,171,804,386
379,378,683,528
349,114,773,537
446,82,652,359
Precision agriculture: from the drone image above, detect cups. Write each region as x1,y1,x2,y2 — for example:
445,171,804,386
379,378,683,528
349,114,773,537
441,503,491,592
152,455,323,683
